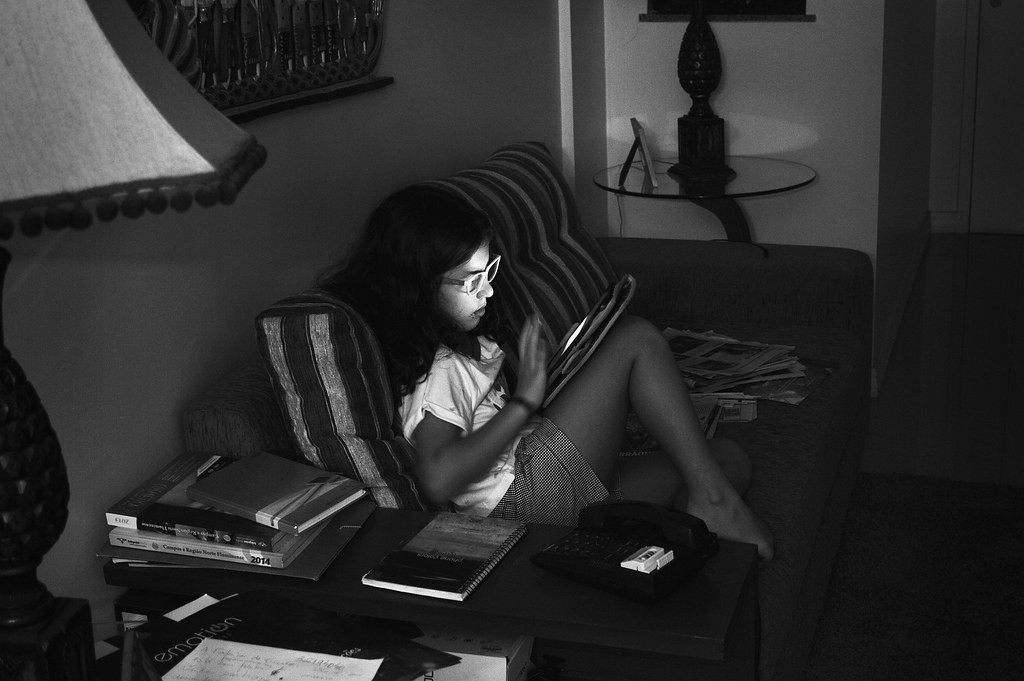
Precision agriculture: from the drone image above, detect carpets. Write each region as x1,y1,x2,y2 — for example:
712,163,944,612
804,472,1024,681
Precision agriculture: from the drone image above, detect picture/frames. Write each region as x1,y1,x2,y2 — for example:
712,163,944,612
619,118,661,186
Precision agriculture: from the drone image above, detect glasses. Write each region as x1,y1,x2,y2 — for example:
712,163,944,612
425,253,501,296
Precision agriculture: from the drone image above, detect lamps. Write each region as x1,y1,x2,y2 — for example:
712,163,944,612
0,0,268,630
637,0,818,177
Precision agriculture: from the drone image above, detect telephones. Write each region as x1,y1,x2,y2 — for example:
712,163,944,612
528,499,721,603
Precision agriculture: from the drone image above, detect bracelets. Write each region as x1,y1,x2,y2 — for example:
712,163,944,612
506,393,537,417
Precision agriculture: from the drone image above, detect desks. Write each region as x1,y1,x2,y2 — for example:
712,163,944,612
0,598,96,681
592,155,817,241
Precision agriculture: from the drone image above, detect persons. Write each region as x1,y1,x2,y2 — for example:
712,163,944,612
344,183,778,567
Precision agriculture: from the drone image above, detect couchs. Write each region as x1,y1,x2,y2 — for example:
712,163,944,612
103,137,874,681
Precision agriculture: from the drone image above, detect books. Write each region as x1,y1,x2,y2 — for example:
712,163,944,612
103,451,380,583
360,511,530,603
